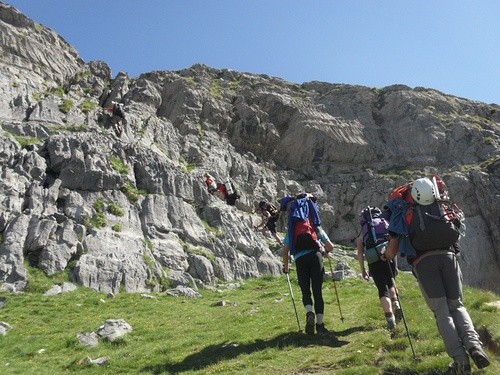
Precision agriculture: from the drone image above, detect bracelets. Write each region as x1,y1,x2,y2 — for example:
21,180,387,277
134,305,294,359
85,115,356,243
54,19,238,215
382,251,389,260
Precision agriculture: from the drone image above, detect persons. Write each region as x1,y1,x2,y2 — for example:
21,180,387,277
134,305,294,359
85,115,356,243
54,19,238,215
203,172,217,196
254,200,285,250
282,193,335,335
101,100,126,138
218,179,236,207
379,180,491,375
357,207,403,330
